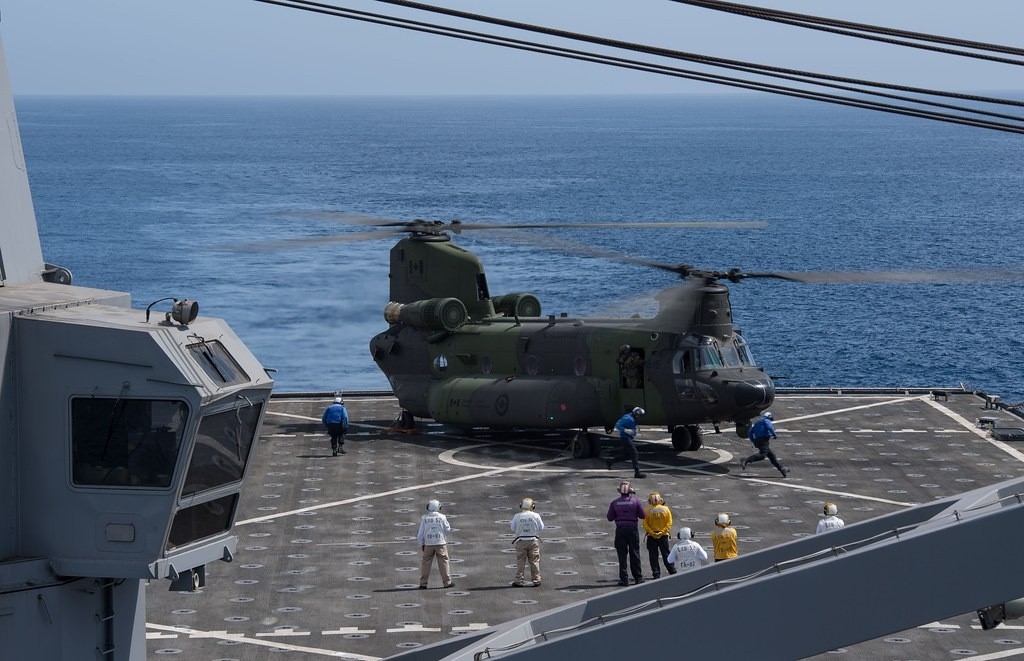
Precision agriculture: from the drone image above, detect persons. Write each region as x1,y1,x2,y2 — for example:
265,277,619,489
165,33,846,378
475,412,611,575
511,497,544,587
322,397,348,456
711,512,739,562
667,527,707,573
740,412,790,478
607,482,647,587
619,344,645,388
816,503,844,535
417,499,455,590
641,492,677,579
607,406,647,479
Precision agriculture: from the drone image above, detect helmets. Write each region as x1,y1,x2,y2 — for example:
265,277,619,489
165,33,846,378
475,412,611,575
522,498,534,510
763,411,774,421
633,407,645,418
334,397,342,404
823,504,838,516
650,493,662,505
428,499,439,511
679,527,692,540
620,481,632,494
717,514,729,526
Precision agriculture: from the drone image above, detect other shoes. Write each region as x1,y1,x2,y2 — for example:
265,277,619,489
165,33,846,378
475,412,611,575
337,447,347,454
443,582,455,588
418,584,428,589
634,473,647,478
606,457,612,470
618,580,628,586
333,450,338,457
635,579,645,584
534,581,542,587
512,582,523,587
782,468,790,477
741,458,747,469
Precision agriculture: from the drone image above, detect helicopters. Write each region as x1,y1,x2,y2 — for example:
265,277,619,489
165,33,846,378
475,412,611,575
230,201,1024,459
